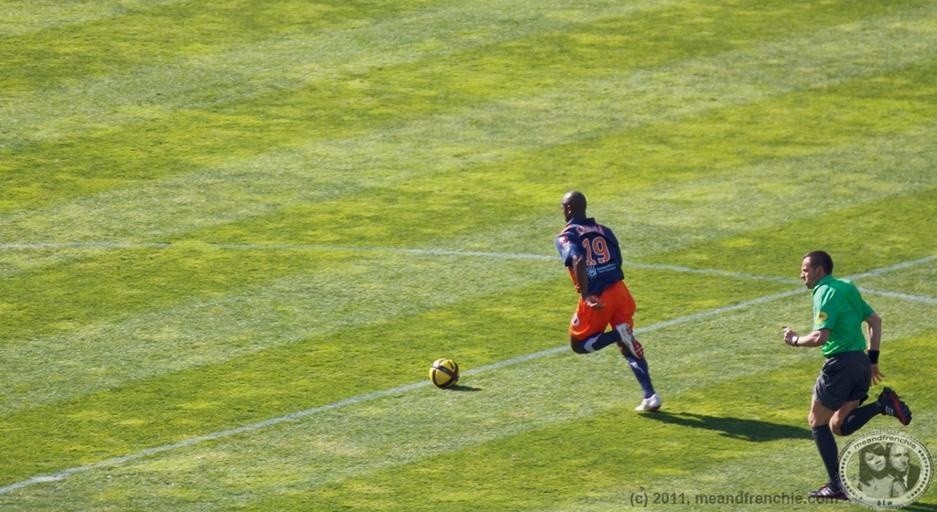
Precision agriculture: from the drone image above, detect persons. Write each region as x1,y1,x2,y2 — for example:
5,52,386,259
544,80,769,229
851,442,921,500
555,191,661,411
784,250,913,500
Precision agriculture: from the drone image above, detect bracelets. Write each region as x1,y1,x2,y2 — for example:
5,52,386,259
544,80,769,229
793,335,798,347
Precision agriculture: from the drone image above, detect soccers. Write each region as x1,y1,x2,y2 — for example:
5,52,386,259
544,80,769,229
429,358,459,388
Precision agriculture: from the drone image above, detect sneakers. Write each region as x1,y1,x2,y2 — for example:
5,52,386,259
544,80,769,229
636,394,661,412
878,387,912,425
809,481,848,500
616,323,644,359
